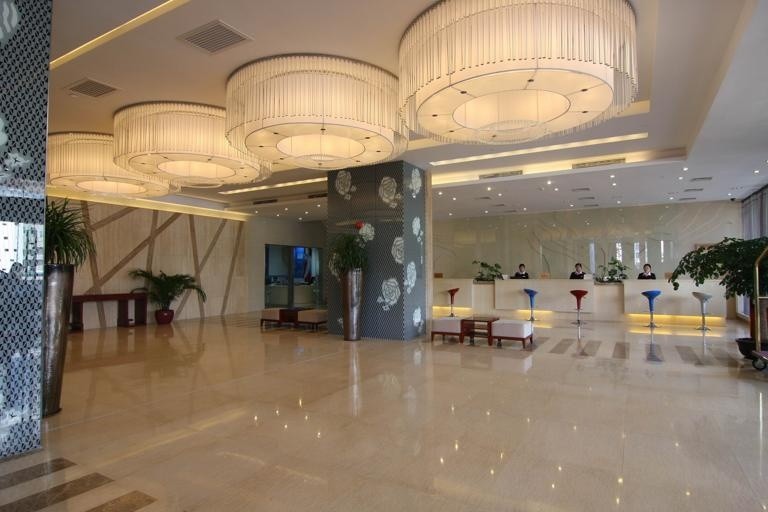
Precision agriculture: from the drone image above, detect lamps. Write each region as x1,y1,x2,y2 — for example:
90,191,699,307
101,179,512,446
224,52,409,171
46,129,184,197
110,100,273,189
397,1,639,149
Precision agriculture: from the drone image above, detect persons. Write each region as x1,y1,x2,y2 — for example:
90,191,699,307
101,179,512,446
569,263,585,279
638,264,656,279
515,264,528,279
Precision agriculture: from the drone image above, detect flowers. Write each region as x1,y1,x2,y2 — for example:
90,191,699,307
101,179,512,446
328,222,376,276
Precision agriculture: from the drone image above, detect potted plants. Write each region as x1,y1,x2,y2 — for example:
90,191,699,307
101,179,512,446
42,198,98,417
665,235,768,358
128,266,209,324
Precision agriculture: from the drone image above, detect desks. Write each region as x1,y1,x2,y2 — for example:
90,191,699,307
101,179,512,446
460,314,501,346
73,291,149,331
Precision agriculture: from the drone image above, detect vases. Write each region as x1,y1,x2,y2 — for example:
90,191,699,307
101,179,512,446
340,265,365,342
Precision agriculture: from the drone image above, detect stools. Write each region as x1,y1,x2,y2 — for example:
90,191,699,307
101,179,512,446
429,313,473,343
297,307,328,331
260,305,296,329
640,289,662,329
691,291,713,331
446,287,460,317
570,289,590,325
523,287,540,321
490,317,534,347
